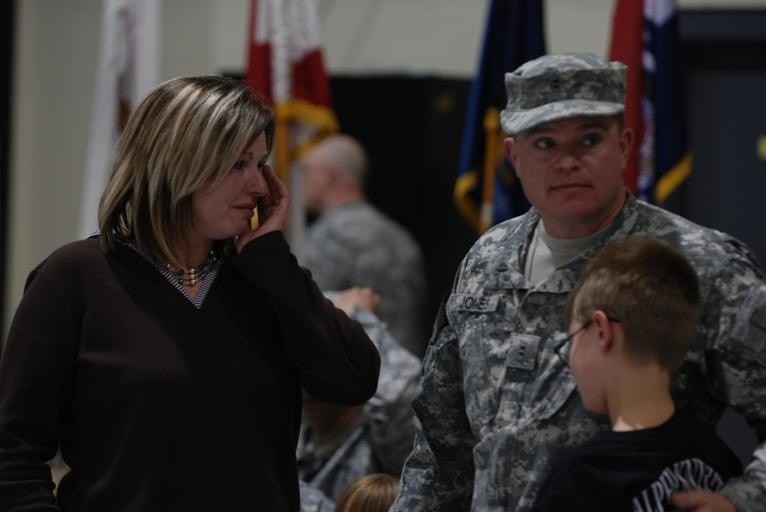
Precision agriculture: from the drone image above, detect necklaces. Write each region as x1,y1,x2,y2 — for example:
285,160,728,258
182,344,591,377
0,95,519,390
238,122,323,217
165,246,220,285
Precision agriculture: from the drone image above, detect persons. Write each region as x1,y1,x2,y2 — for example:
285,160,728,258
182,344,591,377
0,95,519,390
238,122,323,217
531,235,766,512
335,473,400,512
0,76,382,512
389,55,766,512
293,135,426,361
295,286,423,512
454,0,546,236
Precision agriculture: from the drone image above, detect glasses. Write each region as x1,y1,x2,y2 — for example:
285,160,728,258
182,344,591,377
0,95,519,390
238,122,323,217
554,317,621,368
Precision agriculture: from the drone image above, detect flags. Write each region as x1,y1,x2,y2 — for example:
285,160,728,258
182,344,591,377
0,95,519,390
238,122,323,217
608,0,694,219
79,0,162,241
242,0,341,263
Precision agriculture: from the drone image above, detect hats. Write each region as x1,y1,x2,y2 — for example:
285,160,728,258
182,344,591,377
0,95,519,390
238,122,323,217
499,51,627,135
